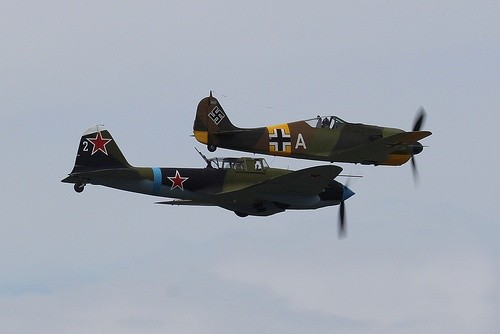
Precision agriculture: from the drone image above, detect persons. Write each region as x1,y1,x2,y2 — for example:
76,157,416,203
321,118,330,128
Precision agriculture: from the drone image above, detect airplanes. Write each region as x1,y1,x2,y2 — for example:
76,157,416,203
191,90,435,192
60,123,356,238
